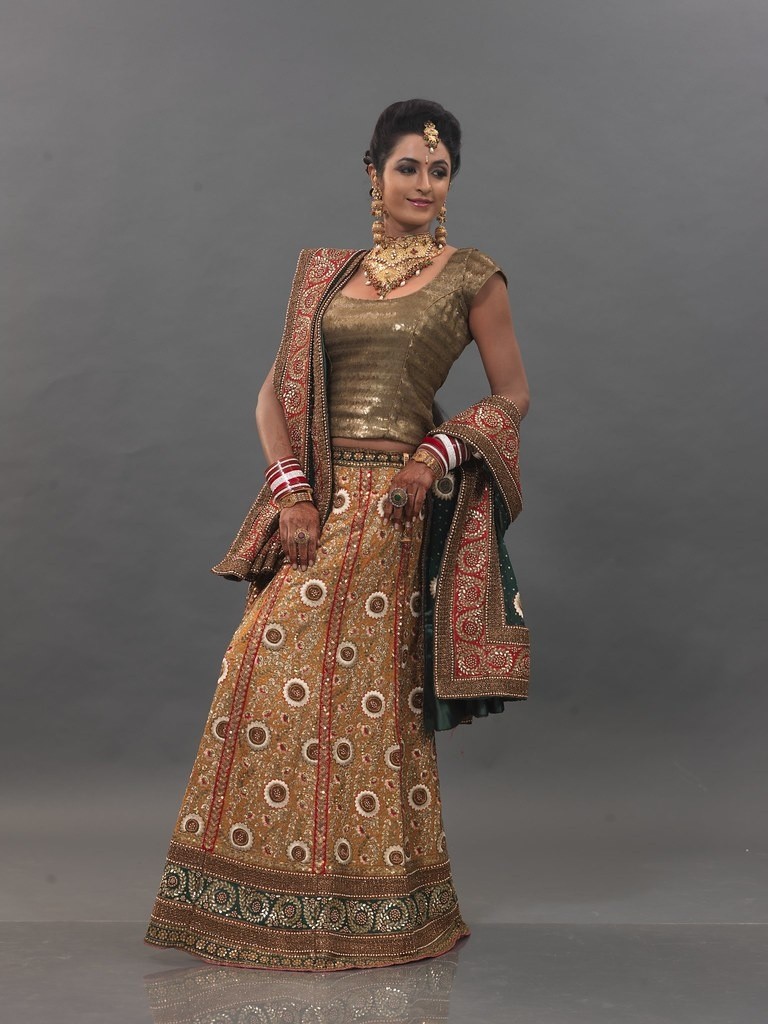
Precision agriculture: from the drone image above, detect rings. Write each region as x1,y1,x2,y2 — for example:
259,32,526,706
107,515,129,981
389,488,407,508
408,494,415,496
293,528,309,544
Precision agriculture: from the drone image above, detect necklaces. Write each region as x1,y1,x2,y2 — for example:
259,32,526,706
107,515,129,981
362,233,445,299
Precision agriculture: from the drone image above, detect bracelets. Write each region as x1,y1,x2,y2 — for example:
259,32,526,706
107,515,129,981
264,455,313,508
411,434,471,477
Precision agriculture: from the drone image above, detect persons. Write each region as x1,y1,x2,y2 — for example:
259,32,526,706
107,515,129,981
141,99,532,973
